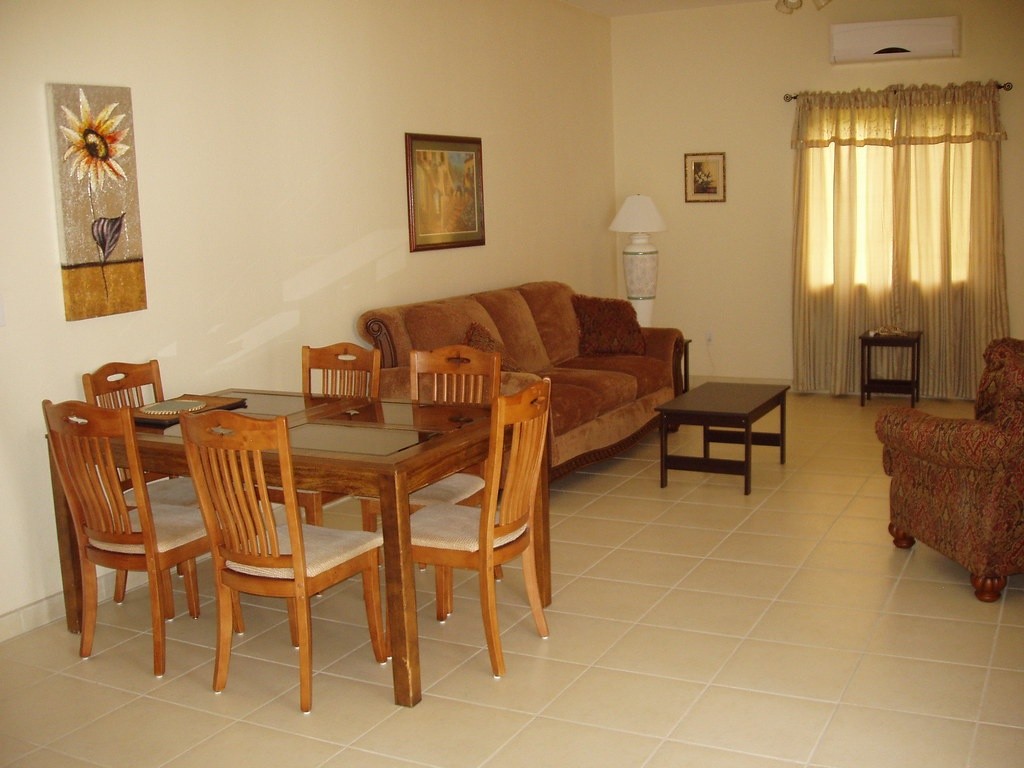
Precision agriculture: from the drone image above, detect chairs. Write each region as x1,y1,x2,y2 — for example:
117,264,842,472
77,359,221,622
179,410,385,714
360,344,501,625
231,342,386,601
40,394,246,679
369,375,554,679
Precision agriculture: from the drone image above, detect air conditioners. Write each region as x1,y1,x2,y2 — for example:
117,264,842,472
831,13,963,63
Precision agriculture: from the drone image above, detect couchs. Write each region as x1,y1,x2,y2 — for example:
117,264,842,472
874,337,1024,603
357,282,692,493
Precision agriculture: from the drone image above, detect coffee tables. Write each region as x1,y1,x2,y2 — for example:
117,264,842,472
653,382,790,495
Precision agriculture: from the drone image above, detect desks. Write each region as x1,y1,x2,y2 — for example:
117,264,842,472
858,330,922,407
50,384,563,707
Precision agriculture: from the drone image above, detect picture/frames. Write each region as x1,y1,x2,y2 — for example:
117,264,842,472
404,132,486,252
684,152,726,205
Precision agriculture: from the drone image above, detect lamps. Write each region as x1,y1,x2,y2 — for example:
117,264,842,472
609,194,666,327
776,0,830,14
